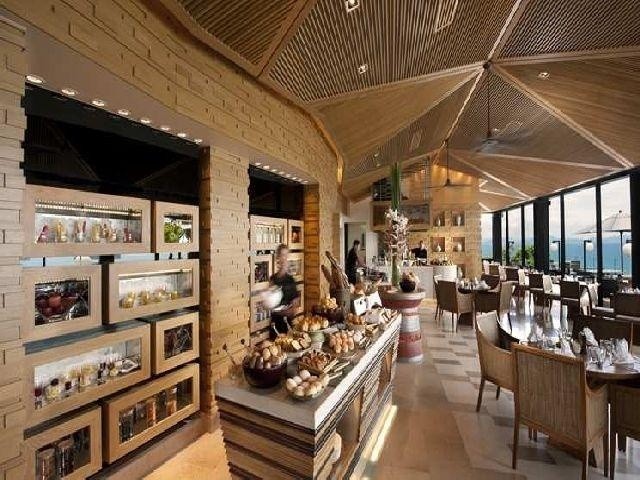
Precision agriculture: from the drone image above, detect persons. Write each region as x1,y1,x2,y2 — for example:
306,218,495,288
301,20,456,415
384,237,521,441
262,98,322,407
412,240,427,265
269,243,300,341
346,240,364,284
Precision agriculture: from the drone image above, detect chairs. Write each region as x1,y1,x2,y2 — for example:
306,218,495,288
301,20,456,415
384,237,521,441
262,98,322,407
570,315,633,357
474,310,520,411
433,259,639,332
511,343,607,478
608,383,640,478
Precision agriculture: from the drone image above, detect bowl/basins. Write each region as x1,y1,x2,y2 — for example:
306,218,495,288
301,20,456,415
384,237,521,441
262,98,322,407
242,359,287,389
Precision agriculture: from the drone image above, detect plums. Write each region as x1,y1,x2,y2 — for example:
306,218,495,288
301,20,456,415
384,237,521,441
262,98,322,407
35,288,76,317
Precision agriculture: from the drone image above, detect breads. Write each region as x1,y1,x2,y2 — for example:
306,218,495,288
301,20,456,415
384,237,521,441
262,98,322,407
320,251,348,289
247,298,366,398
402,272,415,282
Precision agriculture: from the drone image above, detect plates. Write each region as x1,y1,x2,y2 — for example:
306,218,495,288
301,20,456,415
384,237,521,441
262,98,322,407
287,346,313,365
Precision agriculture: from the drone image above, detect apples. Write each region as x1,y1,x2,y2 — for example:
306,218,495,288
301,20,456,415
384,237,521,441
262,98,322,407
165,223,183,243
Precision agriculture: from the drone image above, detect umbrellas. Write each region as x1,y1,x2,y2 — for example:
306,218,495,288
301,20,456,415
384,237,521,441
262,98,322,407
576,210,631,275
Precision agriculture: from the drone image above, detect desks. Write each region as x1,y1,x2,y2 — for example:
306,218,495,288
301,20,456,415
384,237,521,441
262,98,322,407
214,313,402,480
525,337,637,468
378,284,427,363
